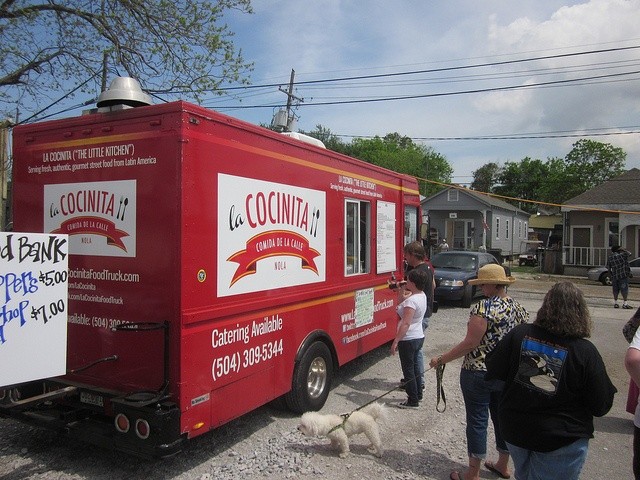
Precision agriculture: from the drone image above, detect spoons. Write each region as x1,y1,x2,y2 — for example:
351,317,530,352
314,209,320,238
121,198,129,221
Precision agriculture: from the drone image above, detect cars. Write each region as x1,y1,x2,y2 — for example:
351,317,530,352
587,257,640,286
428,250,509,307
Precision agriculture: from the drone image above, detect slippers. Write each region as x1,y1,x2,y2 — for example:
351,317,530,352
485,461,510,479
450,471,482,480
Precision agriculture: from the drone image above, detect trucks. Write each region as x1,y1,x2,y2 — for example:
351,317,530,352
12,77,438,462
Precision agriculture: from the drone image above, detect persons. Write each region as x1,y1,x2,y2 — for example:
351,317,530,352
485,281,617,480
625,326,640,479
440,238,448,252
604,246,634,309
390,270,427,410
397,242,433,388
424,248,436,301
429,264,529,479
422,246,437,289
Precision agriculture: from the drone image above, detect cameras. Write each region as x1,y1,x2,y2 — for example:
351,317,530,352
387,272,407,293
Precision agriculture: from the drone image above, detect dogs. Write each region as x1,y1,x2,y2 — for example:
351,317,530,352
296,401,386,458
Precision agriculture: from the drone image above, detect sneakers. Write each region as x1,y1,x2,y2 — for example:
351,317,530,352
614,304,619,308
398,400,418,410
399,383,425,391
407,395,424,402
401,379,406,384
623,303,634,309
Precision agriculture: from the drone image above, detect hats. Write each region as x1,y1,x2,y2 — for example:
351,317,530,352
467,264,510,285
612,245,620,252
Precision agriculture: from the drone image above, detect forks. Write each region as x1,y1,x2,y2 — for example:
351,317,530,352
310,209,316,236
116,197,124,220
